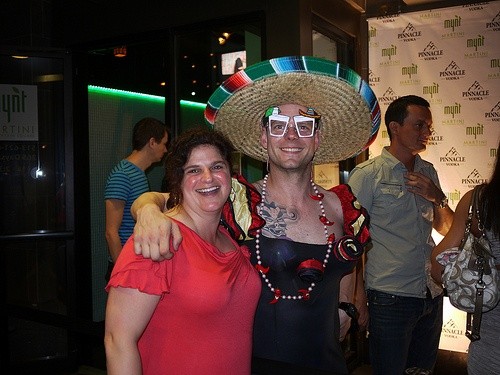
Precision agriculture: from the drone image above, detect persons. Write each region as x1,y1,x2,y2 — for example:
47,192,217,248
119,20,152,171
104,116,168,263
129,54,381,373
431,139,500,375
350,95,454,375
103,126,263,375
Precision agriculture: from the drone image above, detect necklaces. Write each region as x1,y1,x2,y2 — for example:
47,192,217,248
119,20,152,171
253,172,336,305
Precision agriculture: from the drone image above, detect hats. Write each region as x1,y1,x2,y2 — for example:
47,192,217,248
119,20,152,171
203,55,382,163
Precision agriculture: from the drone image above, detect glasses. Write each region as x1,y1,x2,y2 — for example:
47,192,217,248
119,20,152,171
263,114,317,138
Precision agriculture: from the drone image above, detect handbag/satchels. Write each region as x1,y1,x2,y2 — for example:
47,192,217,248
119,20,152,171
436,183,500,342
